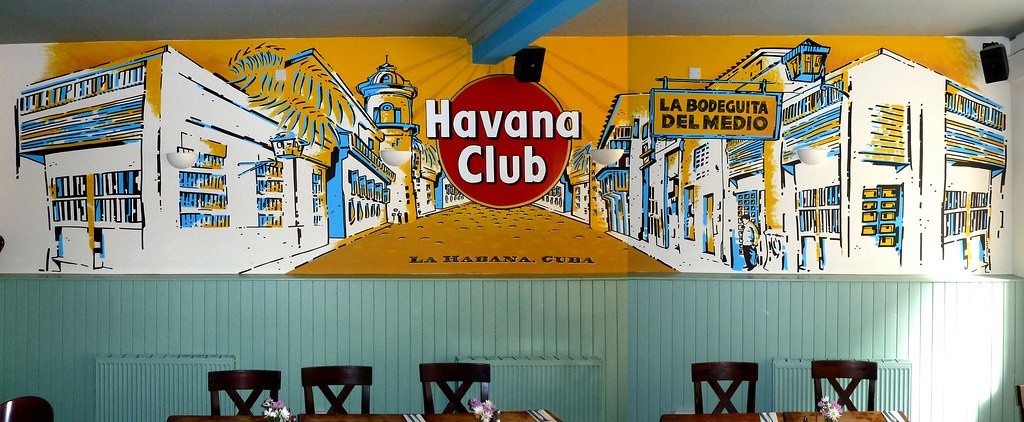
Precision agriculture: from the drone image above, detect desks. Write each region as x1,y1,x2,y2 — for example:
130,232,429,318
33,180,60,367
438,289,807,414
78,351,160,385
783,410,909,422
298,409,564,422
166,416,297,422
659,411,784,422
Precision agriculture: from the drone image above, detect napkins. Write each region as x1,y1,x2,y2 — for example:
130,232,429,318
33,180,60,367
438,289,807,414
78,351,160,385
880,410,905,422
759,412,778,422
402,413,426,422
525,410,556,422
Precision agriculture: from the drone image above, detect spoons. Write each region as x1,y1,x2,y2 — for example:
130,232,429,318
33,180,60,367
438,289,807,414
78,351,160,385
532,410,551,421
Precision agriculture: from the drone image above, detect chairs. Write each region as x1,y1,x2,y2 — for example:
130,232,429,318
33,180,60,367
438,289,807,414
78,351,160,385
811,359,878,412
301,365,373,415
419,363,491,414
1,396,55,422
1017,384,1024,422
690,362,758,414
208,370,281,415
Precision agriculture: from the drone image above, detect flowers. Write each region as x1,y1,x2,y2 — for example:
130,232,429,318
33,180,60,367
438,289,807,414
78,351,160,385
818,396,843,422
261,398,296,422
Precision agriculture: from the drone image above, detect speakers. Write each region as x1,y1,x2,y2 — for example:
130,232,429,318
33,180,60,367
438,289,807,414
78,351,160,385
980,44,1009,84
514,45,546,82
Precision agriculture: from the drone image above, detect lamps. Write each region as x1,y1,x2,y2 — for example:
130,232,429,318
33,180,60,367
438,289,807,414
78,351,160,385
238,131,303,178
165,146,197,170
589,148,624,165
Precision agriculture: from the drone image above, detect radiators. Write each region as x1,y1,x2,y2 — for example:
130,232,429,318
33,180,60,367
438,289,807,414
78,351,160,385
772,357,911,421
455,353,608,421
95,353,237,422
